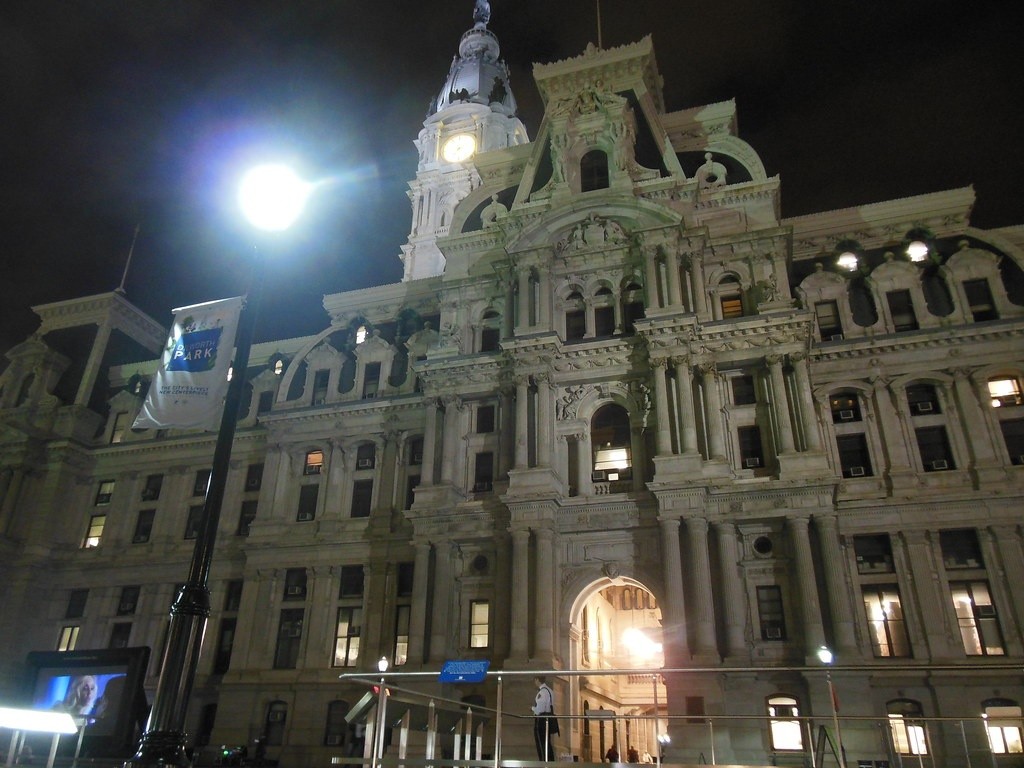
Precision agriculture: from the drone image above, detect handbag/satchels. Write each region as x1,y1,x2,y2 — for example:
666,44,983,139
549,714,559,734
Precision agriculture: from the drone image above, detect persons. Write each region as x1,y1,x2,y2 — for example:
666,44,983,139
642,751,654,764
241,745,247,766
627,746,639,763
46,675,127,736
254,739,267,768
348,722,367,756
606,744,619,762
529,675,560,762
